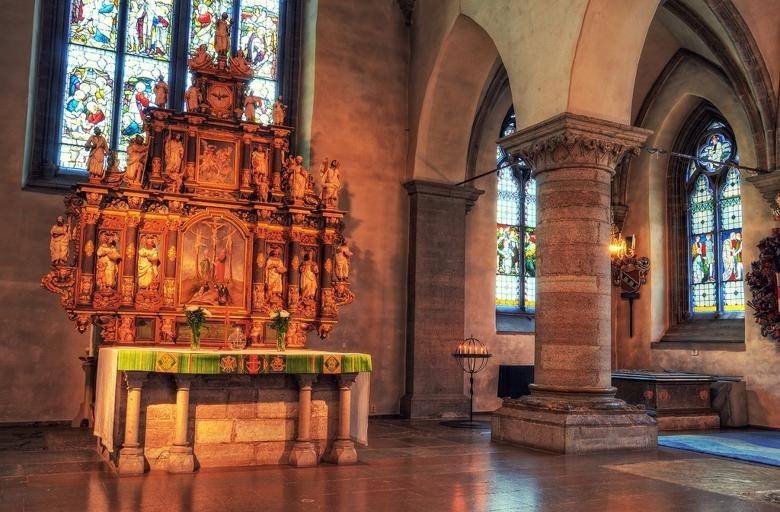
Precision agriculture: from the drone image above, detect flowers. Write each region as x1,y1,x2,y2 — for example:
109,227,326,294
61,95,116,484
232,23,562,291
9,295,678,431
266,308,292,350
181,304,212,349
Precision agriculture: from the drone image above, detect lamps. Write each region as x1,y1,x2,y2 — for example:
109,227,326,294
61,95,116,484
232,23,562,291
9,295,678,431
609,231,637,259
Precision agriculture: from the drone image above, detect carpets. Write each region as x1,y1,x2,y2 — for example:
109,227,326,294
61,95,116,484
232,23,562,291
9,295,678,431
657,429,780,468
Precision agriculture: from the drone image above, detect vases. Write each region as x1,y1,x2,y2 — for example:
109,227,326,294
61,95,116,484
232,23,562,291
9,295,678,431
188,323,202,351
275,328,287,351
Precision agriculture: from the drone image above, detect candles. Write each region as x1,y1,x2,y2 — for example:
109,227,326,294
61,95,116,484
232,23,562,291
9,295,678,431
459,344,488,354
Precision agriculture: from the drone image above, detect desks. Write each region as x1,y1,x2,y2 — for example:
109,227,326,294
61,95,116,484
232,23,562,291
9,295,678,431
93,347,373,474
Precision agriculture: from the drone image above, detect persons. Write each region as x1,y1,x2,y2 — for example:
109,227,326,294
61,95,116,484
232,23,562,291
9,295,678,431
265,248,287,297
212,12,235,55
85,127,109,179
321,159,341,209
333,236,354,283
243,90,263,121
183,80,202,112
250,145,271,202
122,131,186,192
287,155,308,201
299,250,319,300
152,74,170,108
138,239,159,291
272,96,288,125
97,236,121,290
50,215,70,266
191,43,209,64
235,49,251,71
